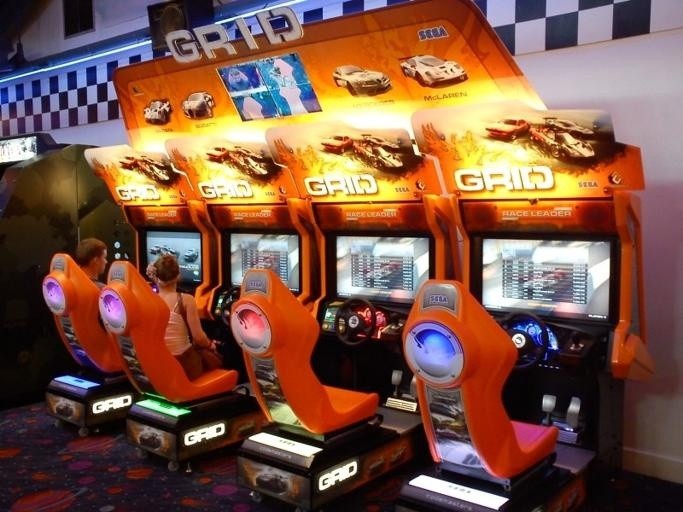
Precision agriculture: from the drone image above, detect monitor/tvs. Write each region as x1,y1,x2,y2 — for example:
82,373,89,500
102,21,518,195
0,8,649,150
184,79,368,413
228,232,301,293
480,236,614,323
147,231,204,289
334,236,430,304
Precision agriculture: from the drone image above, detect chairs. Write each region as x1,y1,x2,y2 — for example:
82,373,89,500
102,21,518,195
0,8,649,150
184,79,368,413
401,280,560,490
98,259,238,406
41,251,122,381
229,267,380,442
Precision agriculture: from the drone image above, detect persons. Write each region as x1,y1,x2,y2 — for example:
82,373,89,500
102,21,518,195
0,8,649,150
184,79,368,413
77,238,108,290
146,254,224,380
229,68,251,92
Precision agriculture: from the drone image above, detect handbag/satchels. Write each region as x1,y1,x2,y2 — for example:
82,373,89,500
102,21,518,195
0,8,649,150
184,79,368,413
202,348,222,370
